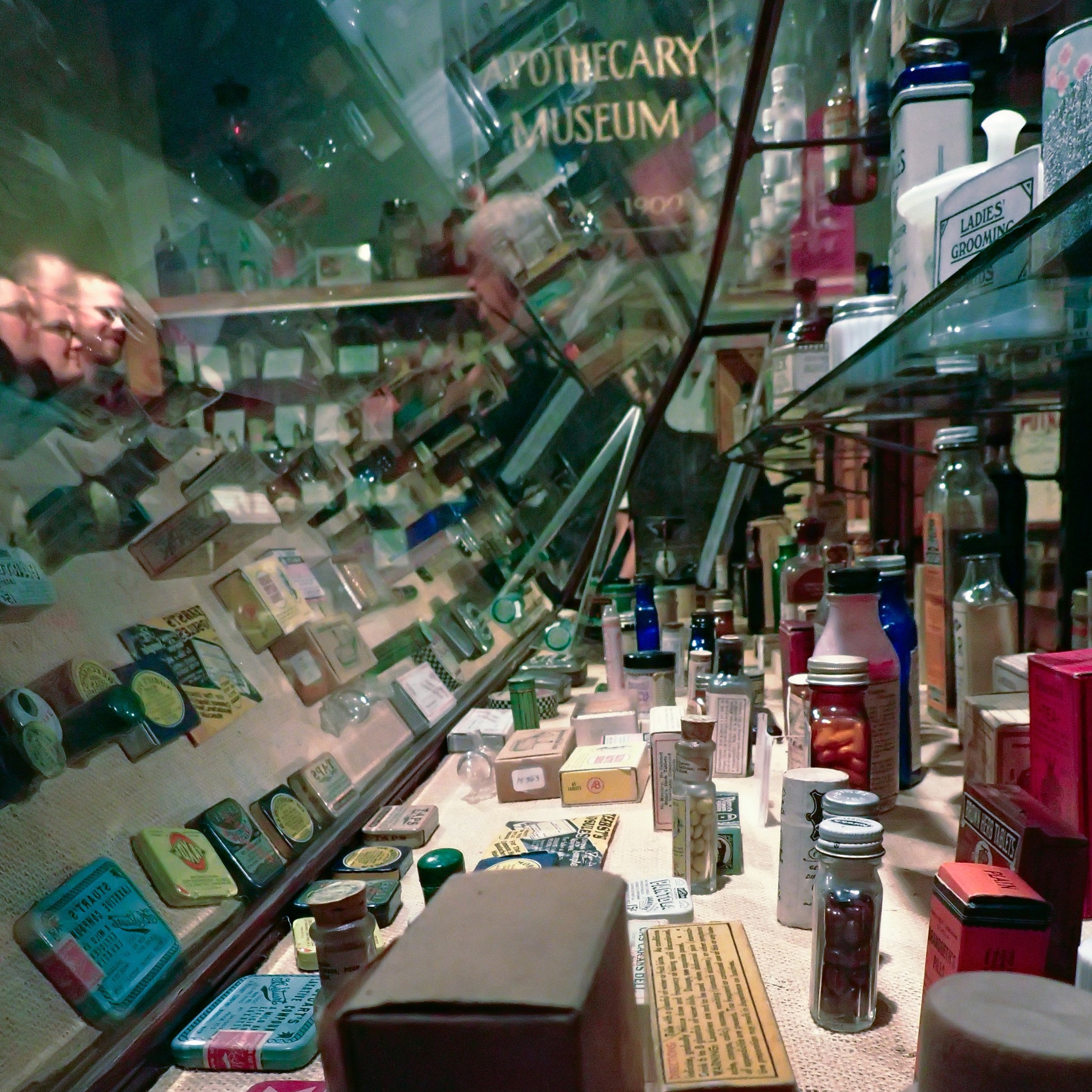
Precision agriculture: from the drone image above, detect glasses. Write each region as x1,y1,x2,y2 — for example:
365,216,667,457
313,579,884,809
41,321,76,340
0,303,29,317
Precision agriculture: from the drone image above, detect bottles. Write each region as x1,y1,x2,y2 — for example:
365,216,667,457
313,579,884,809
152,195,476,298
305,879,381,999
601,12,1092,1092
507,675,540,731
417,847,466,903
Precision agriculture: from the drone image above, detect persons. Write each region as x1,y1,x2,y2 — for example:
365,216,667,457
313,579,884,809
441,192,613,412
0,246,156,416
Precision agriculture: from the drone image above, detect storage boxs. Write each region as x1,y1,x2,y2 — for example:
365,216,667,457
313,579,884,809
558,742,650,807
495,726,577,803
112,653,201,764
135,488,280,582
339,866,643,1092
571,688,637,746
650,706,683,831
955,649,1092,986
212,554,313,654
271,614,379,707
913,863,1051,1079
